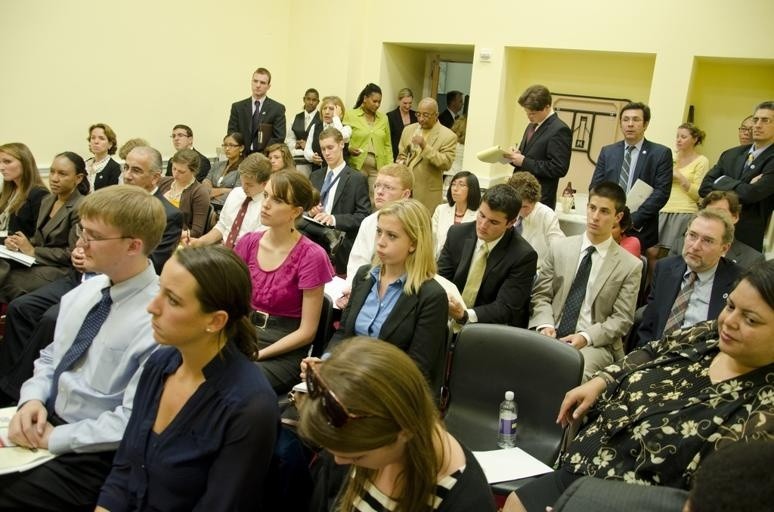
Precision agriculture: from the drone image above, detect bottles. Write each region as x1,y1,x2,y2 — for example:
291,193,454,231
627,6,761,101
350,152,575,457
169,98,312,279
497,391,517,449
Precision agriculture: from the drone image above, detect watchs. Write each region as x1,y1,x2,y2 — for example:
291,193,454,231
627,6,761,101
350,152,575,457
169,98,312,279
591,370,612,387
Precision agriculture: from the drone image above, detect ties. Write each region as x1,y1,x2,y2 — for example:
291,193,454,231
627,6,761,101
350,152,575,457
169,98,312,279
305,116,310,131
452,243,489,334
662,271,697,339
251,101,260,142
742,154,754,169
556,246,596,344
45,287,112,418
224,197,252,249
527,123,538,142
515,216,523,236
618,145,636,193
319,170,333,207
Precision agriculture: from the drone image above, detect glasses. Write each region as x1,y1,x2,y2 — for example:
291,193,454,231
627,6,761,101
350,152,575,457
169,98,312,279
451,184,469,189
415,111,436,118
222,144,242,149
373,184,393,191
751,118,771,126
739,127,752,134
123,164,155,176
685,232,715,247
306,360,375,429
75,223,135,246
170,133,188,138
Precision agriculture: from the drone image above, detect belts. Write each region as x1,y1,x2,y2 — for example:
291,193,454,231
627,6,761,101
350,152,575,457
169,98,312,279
250,310,301,331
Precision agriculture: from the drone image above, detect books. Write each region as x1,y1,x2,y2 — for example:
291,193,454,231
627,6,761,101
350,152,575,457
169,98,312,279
324,275,348,310
0,245,36,267
476,145,512,165
434,274,467,310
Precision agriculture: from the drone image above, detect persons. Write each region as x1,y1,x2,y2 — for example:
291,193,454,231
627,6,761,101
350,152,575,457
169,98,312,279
738,116,757,148
0,151,89,301
0,184,166,512
84,124,120,194
668,190,766,269
120,139,151,161
208,132,246,189
124,147,184,275
303,96,352,170
180,152,272,248
290,89,320,149
232,172,336,395
322,199,448,410
265,144,295,172
509,84,572,210
300,335,498,512
165,125,210,182
645,123,710,298
158,148,210,239
530,183,644,384
438,89,464,129
308,128,372,243
552,442,774,512
95,248,282,512
342,82,393,211
397,96,457,216
610,206,640,257
502,260,774,512
452,95,468,143
698,101,774,254
639,208,745,347
436,183,538,329
227,67,286,155
385,88,418,161
347,161,414,286
590,102,673,308
0,143,49,239
503,173,566,266
430,171,481,265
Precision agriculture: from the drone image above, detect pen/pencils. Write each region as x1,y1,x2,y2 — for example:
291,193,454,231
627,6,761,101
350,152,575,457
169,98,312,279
308,345,314,357
186,235,189,241
514,143,518,150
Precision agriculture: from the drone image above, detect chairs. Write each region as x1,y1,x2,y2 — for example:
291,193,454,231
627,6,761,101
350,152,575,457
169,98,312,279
441,322,584,495
313,293,333,360
